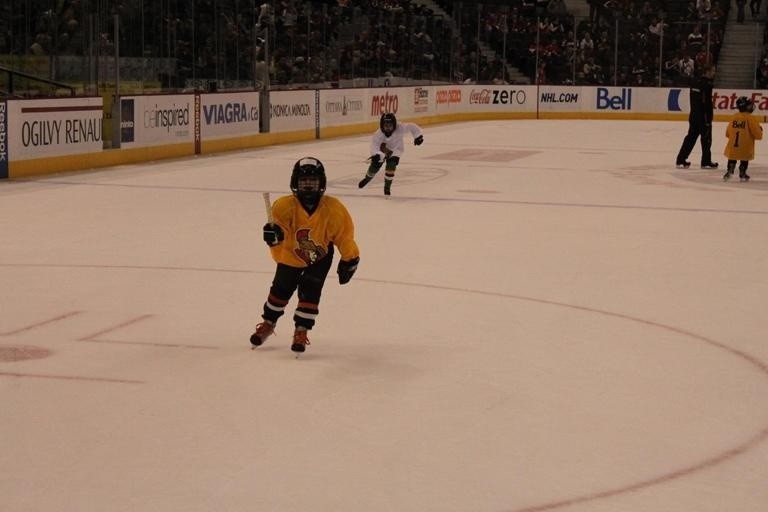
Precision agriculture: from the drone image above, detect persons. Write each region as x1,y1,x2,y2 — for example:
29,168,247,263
675,61,719,168
723,95,764,180
247,155,361,354
356,112,425,196
1,1,768,98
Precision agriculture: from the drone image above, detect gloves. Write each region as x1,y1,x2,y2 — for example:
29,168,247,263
337,257,359,284
371,154,380,163
414,135,423,145
264,222,284,246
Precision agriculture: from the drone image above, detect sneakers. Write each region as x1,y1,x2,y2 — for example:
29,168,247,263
250,320,276,345
677,158,690,166
701,161,718,167
292,325,310,351
359,179,368,188
384,186,390,195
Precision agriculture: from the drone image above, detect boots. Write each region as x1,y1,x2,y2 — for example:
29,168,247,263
725,163,735,177
739,166,750,178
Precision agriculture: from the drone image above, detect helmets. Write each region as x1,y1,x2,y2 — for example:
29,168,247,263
380,112,396,133
290,157,326,202
736,96,754,112
